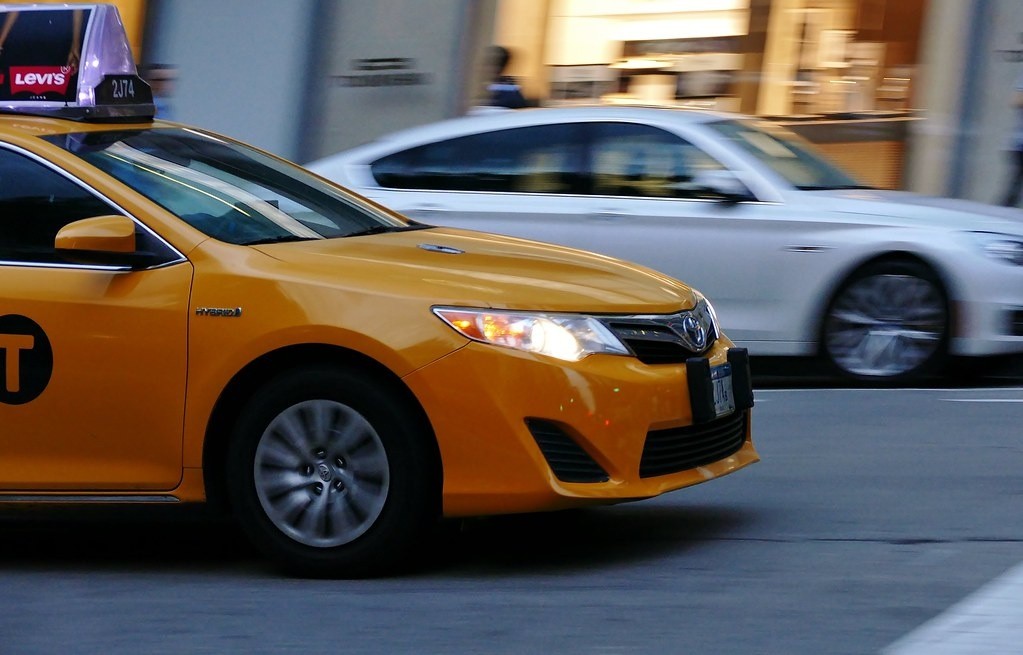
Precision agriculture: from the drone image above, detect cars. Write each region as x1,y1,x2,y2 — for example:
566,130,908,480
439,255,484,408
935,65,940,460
299,104,1023,388
0,118,760,577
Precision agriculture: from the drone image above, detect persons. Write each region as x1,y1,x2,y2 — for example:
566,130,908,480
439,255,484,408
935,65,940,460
993,70,1023,207
144,63,181,121
482,46,528,109
